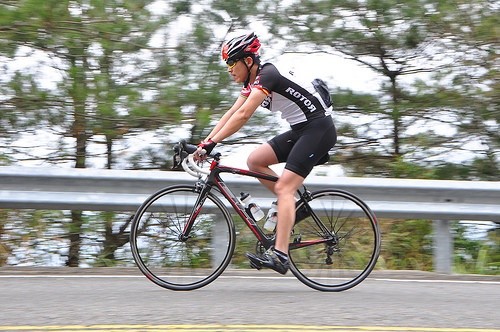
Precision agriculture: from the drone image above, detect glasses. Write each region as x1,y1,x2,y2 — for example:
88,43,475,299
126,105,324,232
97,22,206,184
225,57,242,68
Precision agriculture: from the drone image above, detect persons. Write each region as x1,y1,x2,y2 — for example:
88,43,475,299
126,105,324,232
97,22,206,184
195,31,337,275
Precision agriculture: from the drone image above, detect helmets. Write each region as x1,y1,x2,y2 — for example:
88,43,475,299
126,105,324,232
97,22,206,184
220,31,261,63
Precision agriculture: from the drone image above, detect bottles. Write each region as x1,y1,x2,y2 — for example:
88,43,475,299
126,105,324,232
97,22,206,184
238,191,265,222
263,199,279,231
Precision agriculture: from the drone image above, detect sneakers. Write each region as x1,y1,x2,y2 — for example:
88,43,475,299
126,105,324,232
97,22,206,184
295,199,310,225
245,245,290,275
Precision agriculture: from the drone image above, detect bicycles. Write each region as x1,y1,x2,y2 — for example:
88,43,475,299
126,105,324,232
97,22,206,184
129,142,383,292
